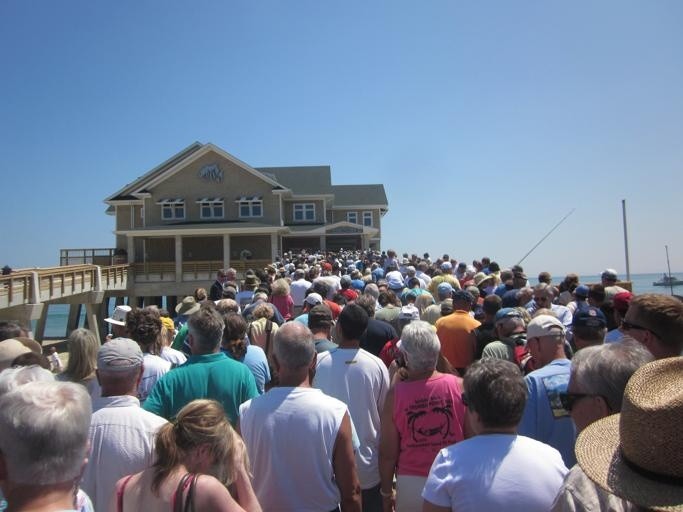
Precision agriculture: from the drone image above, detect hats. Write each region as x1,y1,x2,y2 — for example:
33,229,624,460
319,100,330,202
600,269,618,282
440,262,453,271
174,296,202,316
474,271,493,288
566,305,609,329
575,285,590,296
491,307,523,337
340,275,352,287
398,305,420,321
96,337,144,371
572,356,683,511
608,291,634,305
241,274,259,288
294,268,304,277
0,336,44,371
302,292,323,305
523,314,566,354
104,304,133,327
438,282,456,296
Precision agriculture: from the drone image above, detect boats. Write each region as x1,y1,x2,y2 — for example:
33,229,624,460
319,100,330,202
653,276,683,287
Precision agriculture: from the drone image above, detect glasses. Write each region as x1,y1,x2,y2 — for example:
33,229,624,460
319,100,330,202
533,296,548,302
559,388,614,413
619,318,663,340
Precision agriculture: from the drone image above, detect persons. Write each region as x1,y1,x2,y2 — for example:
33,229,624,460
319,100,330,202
2,248,682,512
